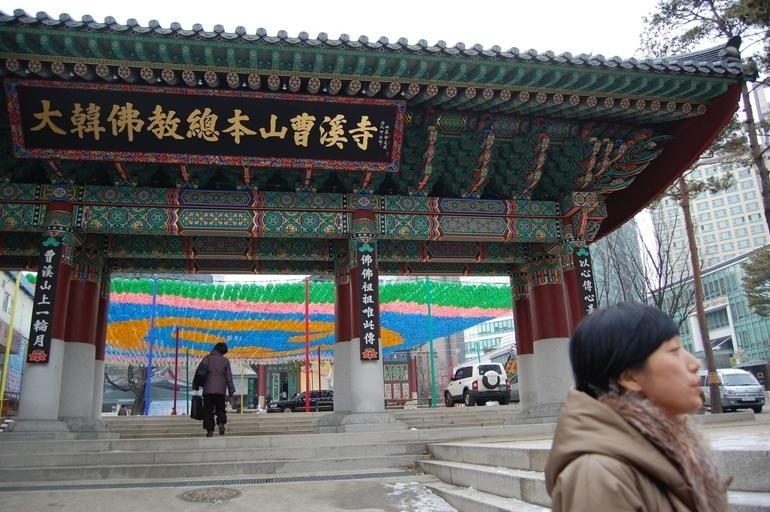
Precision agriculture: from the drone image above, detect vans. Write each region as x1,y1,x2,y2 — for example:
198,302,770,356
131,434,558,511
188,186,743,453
696,368,766,414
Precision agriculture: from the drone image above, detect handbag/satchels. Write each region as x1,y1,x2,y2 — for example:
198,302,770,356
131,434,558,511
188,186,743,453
196,367,207,386
190,396,203,420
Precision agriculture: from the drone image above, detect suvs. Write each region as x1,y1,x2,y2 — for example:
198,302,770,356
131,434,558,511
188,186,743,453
444,362,510,407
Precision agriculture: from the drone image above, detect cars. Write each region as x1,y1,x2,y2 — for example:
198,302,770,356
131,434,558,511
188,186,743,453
509,373,520,402
0,418,15,434
266,390,333,414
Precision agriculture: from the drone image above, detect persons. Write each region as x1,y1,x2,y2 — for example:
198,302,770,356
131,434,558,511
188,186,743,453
192,342,236,437
544,301,733,510
